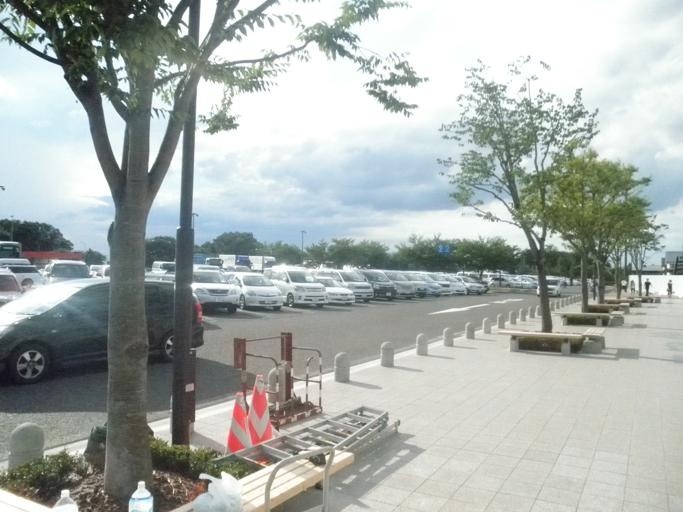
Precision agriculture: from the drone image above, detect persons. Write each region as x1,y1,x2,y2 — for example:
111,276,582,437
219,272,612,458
620,278,626,291
643,277,651,296
666,279,673,298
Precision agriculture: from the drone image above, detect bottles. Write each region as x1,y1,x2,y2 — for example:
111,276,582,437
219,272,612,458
51,488,78,511
128,480,154,512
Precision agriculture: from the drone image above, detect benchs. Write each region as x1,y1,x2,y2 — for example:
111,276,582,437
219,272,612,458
497,292,663,358
170,442,363,512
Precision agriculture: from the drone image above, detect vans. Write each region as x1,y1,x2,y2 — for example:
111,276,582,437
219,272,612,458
0,277,204,384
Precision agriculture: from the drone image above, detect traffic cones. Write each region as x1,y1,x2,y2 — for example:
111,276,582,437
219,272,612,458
220,392,253,458
245,373,272,445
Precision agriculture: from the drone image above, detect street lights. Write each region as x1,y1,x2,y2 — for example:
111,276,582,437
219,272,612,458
189,212,197,228
300,230,306,254
